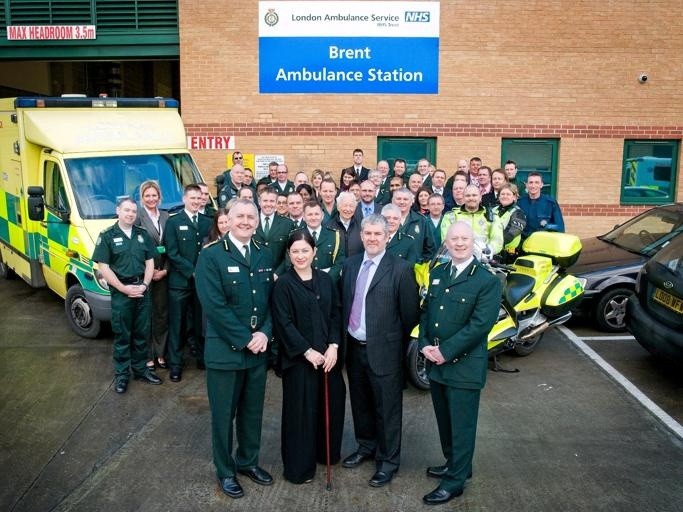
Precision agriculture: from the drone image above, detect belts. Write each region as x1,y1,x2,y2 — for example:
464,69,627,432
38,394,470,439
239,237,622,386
346,331,367,347
120,273,144,285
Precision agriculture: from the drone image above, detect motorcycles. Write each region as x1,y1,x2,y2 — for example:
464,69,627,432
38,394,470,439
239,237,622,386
409,232,584,390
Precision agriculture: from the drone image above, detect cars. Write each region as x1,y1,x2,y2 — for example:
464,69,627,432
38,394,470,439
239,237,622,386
620,231,682,375
625,188,669,198
559,201,682,334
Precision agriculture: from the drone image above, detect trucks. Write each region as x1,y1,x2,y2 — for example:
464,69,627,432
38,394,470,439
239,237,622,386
626,156,673,192
1,89,217,344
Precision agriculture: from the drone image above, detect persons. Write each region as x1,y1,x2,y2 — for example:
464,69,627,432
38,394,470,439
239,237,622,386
417,222,502,506
194,195,273,497
273,233,345,484
341,212,419,486
132,150,564,383
93,198,162,394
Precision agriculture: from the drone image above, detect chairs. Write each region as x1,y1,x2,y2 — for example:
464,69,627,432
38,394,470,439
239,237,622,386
122,162,159,200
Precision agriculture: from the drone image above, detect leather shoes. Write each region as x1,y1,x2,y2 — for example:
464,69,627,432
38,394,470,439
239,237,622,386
145,359,157,372
156,357,168,369
366,464,403,489
422,482,465,506
341,451,374,470
114,375,129,395
169,365,185,382
132,371,162,386
215,473,245,500
426,465,457,479
237,464,275,485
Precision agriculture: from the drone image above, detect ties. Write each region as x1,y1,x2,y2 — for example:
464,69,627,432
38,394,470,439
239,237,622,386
346,258,376,336
191,215,198,230
292,219,299,230
264,216,271,235
481,188,486,195
449,265,459,283
312,230,319,246
356,168,360,177
435,189,440,194
363,207,373,220
242,244,252,268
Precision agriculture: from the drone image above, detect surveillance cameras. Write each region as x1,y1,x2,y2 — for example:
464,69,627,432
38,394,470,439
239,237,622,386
639,74,647,82
